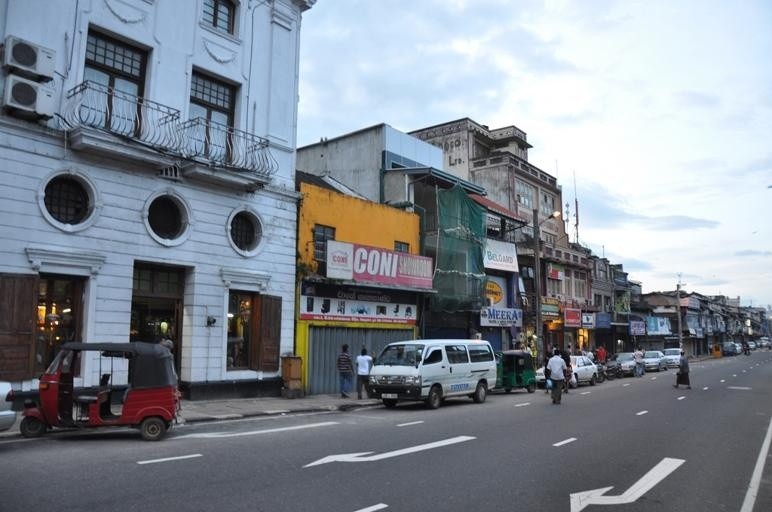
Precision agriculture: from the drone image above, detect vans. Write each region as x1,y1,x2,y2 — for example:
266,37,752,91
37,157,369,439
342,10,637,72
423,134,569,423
367,339,497,409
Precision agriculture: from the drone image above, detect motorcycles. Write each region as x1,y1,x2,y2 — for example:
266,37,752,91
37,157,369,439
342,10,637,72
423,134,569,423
20,341,182,441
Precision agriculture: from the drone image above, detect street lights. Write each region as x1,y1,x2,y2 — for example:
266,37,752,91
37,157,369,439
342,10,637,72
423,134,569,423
533,208,561,365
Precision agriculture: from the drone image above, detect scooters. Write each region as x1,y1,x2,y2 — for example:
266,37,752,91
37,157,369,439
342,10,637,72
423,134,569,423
495,350,537,394
592,360,626,383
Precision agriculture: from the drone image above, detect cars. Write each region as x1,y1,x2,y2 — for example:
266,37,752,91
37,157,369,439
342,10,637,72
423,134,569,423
0,381,16,431
535,355,599,388
722,336,769,356
641,351,668,371
661,348,684,368
606,352,645,377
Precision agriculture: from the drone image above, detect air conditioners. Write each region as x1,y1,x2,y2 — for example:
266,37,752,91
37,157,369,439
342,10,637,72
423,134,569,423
3,34,56,83
3,74,54,120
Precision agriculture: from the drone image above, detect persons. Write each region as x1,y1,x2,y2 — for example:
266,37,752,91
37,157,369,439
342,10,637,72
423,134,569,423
355,348,375,400
633,348,644,379
158,340,178,387
759,339,764,351
709,341,713,355
767,339,772,350
743,341,747,355
672,351,691,389
336,343,354,399
747,341,751,354
640,346,646,373
546,350,567,405
519,341,609,394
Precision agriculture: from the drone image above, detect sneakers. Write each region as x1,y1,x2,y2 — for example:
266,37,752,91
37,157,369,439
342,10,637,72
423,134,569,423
341,390,350,400
